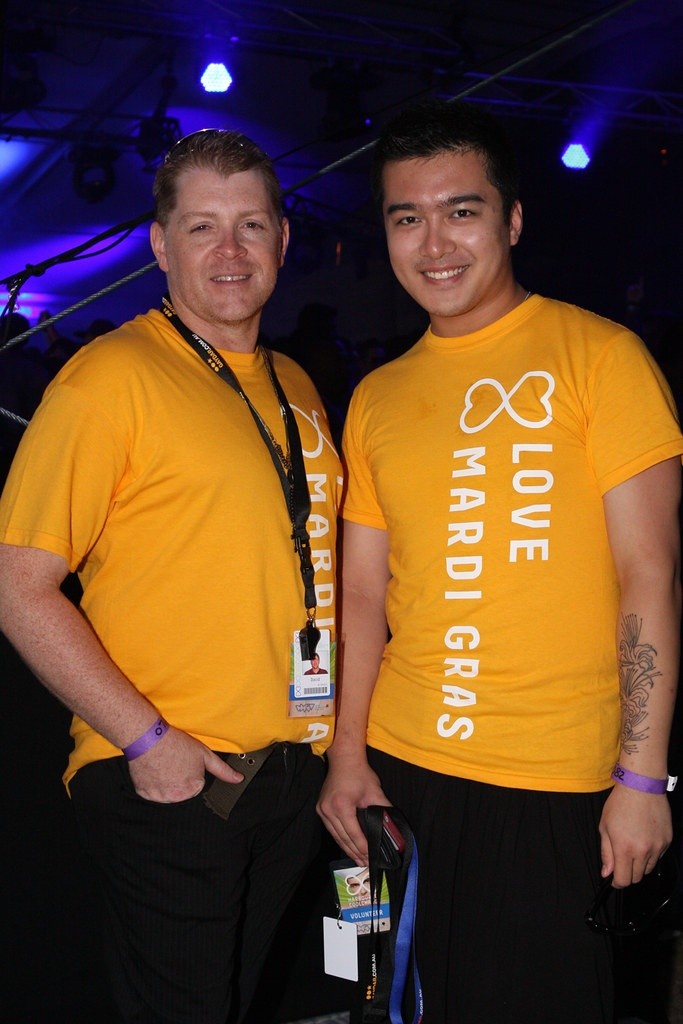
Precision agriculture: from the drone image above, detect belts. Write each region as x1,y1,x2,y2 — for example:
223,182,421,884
205,744,277,821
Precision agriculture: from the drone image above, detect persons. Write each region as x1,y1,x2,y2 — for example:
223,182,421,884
334,867,377,905
0,129,347,1024
306,653,328,675
315,97,683,1024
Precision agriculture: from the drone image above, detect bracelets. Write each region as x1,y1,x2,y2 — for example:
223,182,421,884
123,715,169,761
611,763,678,795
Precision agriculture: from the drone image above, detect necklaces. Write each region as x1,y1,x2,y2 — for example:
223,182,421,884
525,291,531,300
